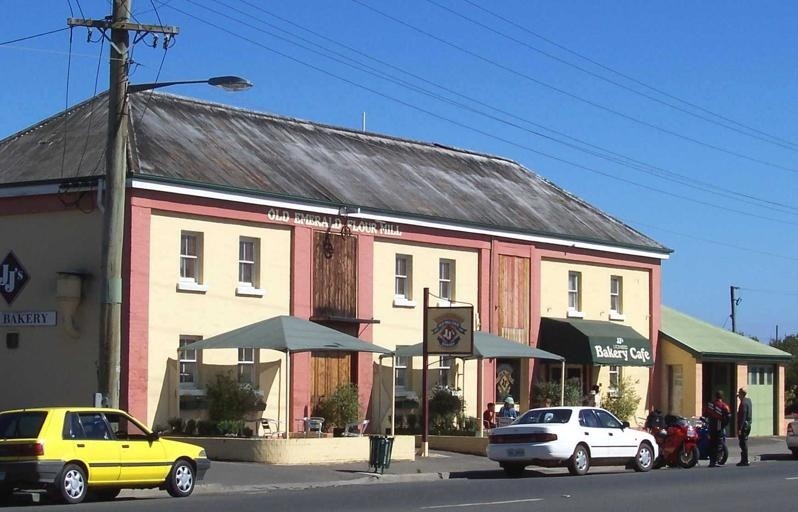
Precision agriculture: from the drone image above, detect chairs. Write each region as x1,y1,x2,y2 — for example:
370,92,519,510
304,416,326,438
343,419,369,437
260,418,285,438
459,415,514,432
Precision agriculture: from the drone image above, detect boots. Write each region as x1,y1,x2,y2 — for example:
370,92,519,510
735,451,751,467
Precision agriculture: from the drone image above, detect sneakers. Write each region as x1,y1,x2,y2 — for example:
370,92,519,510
707,461,725,468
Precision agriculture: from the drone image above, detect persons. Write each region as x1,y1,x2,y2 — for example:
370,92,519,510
539,397,552,407
499,397,517,418
702,389,732,466
483,402,497,428
735,386,753,466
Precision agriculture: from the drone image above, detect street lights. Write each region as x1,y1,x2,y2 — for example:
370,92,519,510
100,73,250,419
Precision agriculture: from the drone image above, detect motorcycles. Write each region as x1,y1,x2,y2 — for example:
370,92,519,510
686,411,729,466
641,410,700,470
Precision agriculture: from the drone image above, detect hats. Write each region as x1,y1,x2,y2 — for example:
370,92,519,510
735,388,747,396
504,396,515,405
713,390,726,400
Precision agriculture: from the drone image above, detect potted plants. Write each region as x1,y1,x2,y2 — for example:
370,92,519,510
313,383,361,438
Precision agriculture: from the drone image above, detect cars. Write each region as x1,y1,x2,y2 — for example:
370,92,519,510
484,406,660,472
783,420,797,453
1,406,211,503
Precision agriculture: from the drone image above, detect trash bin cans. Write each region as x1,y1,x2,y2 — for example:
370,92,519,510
369,434,394,474
